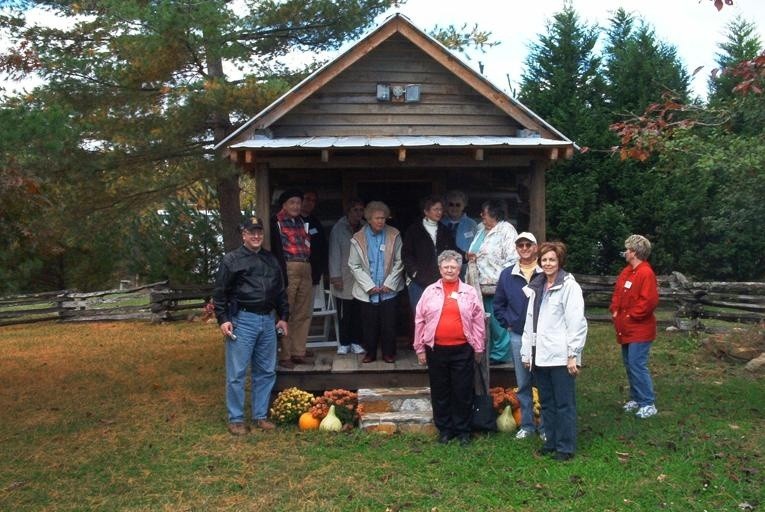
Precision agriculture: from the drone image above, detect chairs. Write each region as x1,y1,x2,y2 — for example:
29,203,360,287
306,272,343,348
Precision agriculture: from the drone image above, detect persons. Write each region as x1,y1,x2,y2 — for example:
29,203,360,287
519,240,588,463
609,235,662,420
405,188,479,362
493,231,548,441
414,249,486,445
269,184,326,366
212,218,289,436
328,195,408,363
471,199,518,365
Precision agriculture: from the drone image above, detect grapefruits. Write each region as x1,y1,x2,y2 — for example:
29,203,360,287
298,412,320,432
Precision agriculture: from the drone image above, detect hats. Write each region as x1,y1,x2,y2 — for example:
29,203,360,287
514,232,537,245
278,190,304,205
244,217,264,231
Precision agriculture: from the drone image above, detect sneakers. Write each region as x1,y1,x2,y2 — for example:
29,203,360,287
279,360,295,369
439,433,454,443
488,357,507,366
336,345,351,355
226,420,247,436
459,433,474,446
516,428,536,439
623,399,657,420
350,342,366,354
252,418,275,431
291,355,313,365
539,433,575,461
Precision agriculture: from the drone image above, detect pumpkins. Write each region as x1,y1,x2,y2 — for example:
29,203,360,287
496,405,517,432
319,404,342,432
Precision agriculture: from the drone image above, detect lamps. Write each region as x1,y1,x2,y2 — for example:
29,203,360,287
375,81,422,104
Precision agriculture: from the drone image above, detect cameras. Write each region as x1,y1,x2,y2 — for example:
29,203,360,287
276,328,284,337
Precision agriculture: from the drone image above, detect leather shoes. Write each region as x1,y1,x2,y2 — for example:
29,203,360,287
362,352,376,363
382,353,394,363
